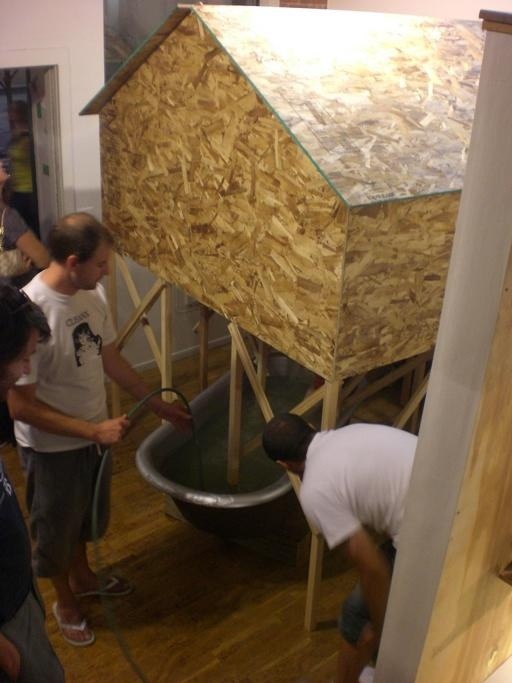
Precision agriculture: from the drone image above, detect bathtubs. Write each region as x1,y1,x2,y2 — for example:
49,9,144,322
136,352,341,542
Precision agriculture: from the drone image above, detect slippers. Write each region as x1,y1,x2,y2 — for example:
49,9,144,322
74,573,134,598
53,600,95,646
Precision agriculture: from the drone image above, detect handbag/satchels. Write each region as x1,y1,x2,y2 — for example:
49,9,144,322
1,248,33,278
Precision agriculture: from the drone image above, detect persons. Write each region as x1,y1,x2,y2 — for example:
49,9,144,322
262,413,418,683
6,213,194,649
0,282,68,683
0,152,49,288
